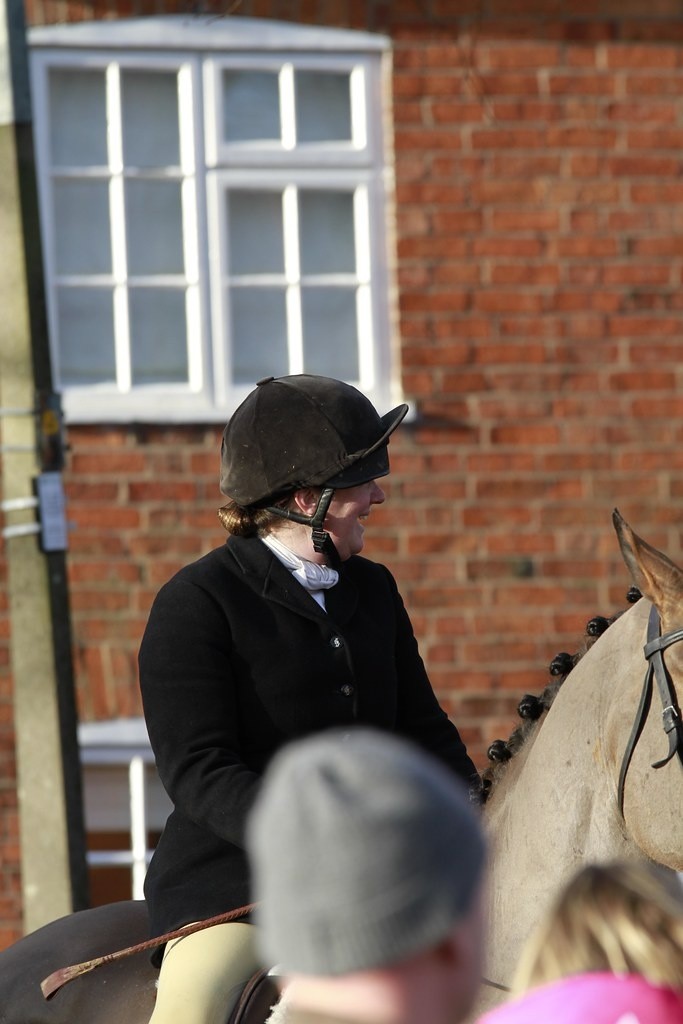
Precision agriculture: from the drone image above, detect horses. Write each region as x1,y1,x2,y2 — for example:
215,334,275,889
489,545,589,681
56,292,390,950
0,506,683,1023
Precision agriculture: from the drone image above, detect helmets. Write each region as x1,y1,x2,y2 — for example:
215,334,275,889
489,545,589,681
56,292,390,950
220,374,409,508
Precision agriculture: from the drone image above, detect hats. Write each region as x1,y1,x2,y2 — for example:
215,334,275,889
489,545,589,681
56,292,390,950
247,732,487,975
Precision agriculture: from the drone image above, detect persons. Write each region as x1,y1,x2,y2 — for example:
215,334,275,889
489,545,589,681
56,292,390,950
246,733,682,1023
136,374,484,1023
510,863,683,997
